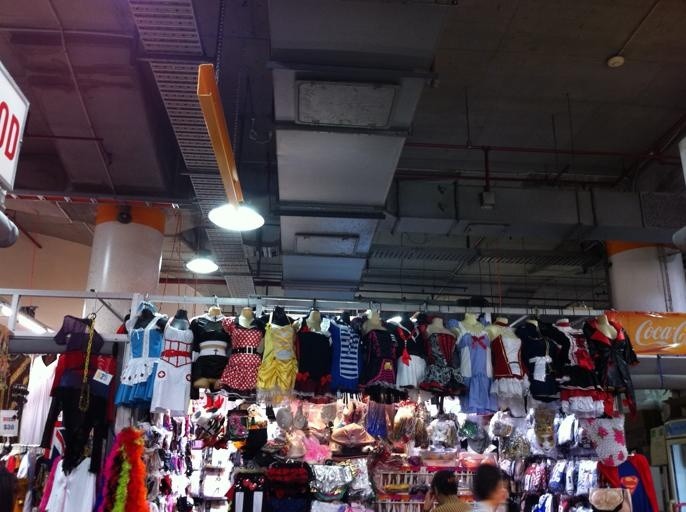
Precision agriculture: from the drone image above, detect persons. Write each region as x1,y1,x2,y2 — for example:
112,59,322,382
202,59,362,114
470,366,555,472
467,462,511,512
420,469,471,511
115,301,641,420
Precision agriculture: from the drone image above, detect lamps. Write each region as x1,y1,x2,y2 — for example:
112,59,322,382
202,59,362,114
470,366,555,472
206,74,266,234
185,213,219,275
